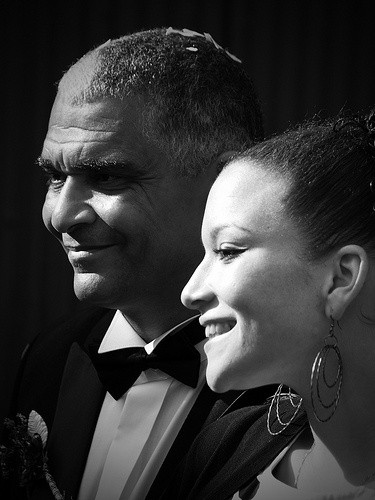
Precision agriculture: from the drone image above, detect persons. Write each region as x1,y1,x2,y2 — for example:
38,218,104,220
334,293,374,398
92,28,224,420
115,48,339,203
179,111,375,500
1,27,308,500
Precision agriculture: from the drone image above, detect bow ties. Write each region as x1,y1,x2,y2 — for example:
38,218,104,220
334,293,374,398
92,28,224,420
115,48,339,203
89,317,207,401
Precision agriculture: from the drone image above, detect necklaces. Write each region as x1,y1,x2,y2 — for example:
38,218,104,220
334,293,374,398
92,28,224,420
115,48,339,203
294,443,375,489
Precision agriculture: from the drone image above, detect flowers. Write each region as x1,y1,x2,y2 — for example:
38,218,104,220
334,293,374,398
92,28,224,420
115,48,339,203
0,406,49,486
